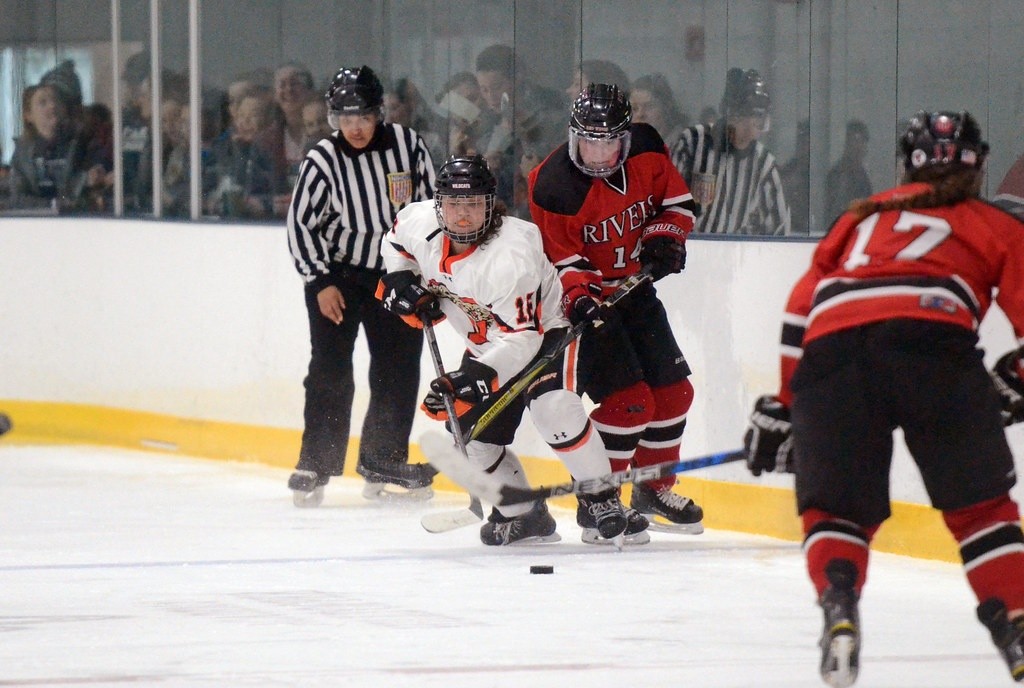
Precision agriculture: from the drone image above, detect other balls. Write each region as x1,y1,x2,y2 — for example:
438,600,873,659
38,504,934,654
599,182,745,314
530,565,554,574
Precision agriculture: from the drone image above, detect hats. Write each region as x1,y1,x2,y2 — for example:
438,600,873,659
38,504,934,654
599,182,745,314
39,60,82,102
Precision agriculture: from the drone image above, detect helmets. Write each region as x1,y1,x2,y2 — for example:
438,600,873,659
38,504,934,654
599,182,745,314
719,69,771,116
324,65,383,117
433,155,497,244
569,83,633,176
899,108,989,173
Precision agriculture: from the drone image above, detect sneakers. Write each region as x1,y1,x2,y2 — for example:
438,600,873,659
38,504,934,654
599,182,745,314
818,557,861,688
977,597,1024,681
575,494,650,547
586,484,628,554
289,467,329,508
481,499,561,546
356,427,432,503
630,479,706,535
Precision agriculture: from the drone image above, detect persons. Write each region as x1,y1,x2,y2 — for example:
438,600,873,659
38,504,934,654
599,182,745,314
383,43,570,222
526,83,704,536
286,65,439,488
0,56,333,223
666,66,790,235
381,155,627,546
745,107,1024,688
627,73,690,146
565,58,630,99
826,119,875,232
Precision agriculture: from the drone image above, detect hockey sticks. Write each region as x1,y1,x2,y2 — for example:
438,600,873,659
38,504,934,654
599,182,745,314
417,428,748,507
359,262,654,481
420,313,484,534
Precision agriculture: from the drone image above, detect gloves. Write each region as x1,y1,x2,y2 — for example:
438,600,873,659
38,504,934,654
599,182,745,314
420,359,500,421
637,235,686,282
744,396,795,475
374,270,448,329
570,296,616,340
988,345,1024,425
